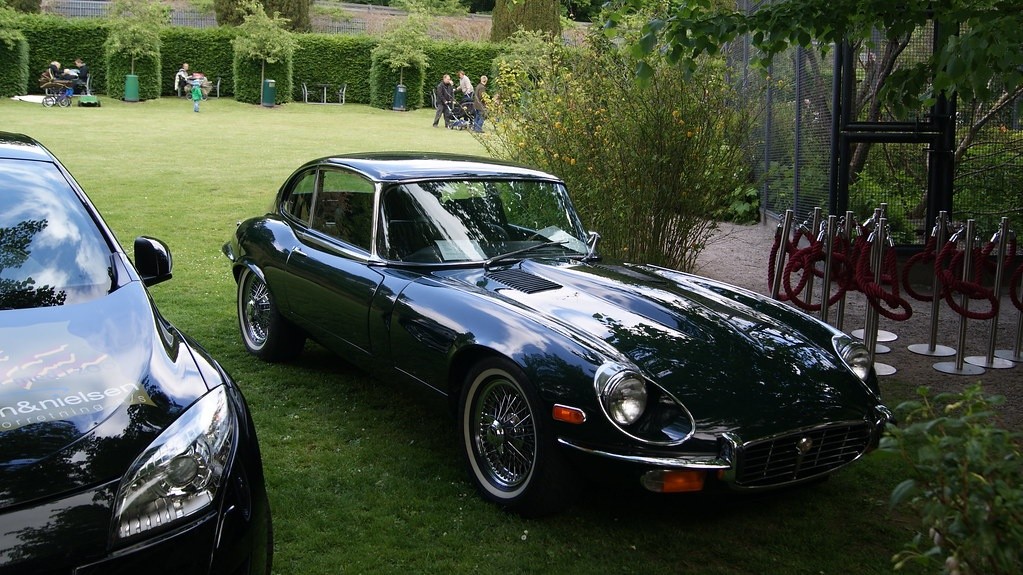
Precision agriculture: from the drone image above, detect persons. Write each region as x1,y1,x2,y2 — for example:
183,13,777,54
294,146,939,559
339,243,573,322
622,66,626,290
474,75,488,133
433,74,453,127
179,62,192,87
72,58,88,95
456,70,475,125
51,60,80,77
191,80,203,112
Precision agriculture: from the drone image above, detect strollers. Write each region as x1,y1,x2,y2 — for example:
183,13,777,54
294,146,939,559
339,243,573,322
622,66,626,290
38,67,76,107
445,101,476,130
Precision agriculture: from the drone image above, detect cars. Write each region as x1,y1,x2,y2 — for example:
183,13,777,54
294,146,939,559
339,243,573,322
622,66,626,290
1,125,279,575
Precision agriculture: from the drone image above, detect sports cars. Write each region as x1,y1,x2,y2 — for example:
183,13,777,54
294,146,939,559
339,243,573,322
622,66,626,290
221,150,894,523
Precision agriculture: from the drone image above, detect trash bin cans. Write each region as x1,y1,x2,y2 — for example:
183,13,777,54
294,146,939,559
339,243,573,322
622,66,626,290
124,75,139,102
394,84,407,109
262,78,276,107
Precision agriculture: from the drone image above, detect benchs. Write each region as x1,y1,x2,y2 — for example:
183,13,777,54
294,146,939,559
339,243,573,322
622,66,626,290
289,193,376,242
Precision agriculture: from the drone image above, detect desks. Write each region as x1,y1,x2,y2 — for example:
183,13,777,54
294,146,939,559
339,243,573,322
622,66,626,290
317,83,333,104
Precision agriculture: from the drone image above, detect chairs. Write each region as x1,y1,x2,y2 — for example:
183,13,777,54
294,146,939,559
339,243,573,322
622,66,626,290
73,73,92,97
331,83,348,105
388,199,442,259
446,194,521,242
300,83,318,104
175,72,192,99
429,89,438,110
207,77,221,99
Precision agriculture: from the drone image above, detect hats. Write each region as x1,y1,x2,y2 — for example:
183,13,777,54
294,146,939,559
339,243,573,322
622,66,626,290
193,80,199,85
